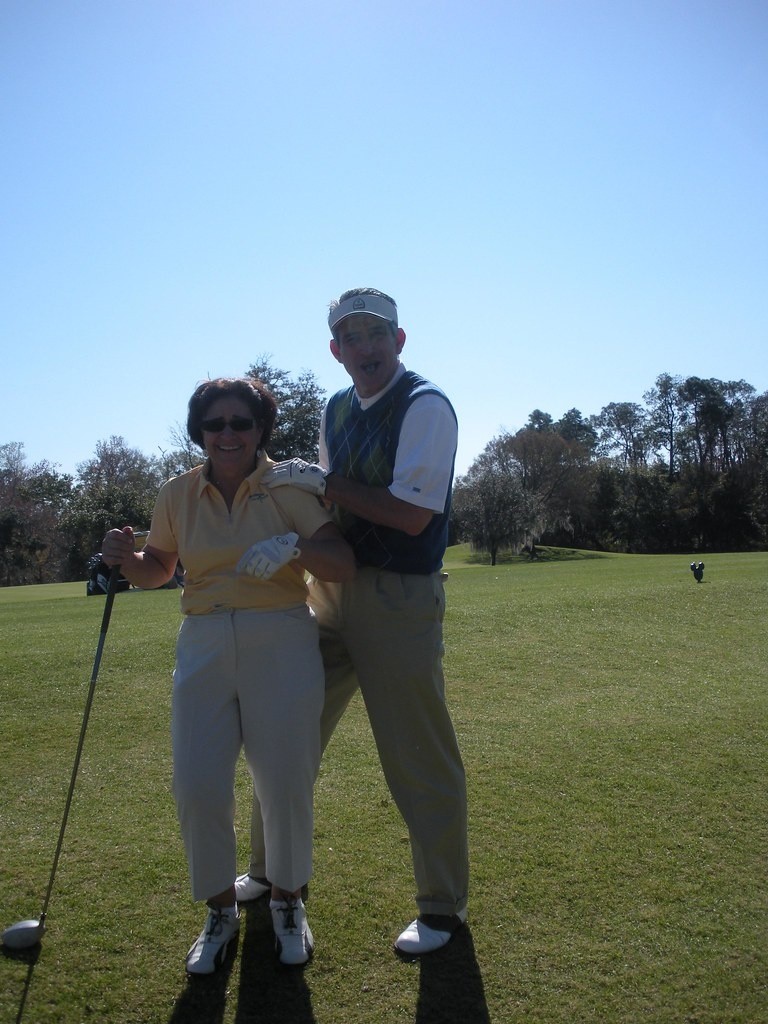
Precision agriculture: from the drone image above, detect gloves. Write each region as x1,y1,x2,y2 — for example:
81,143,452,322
260,458,334,497
236,532,301,581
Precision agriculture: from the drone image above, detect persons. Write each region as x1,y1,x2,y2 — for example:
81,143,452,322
232,287,469,959
101,378,355,975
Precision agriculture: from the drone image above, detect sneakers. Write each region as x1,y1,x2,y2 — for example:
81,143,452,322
185,901,242,974
234,872,270,902
394,906,466,955
269,893,315,964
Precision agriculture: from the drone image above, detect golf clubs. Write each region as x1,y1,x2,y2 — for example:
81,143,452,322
2,563,122,951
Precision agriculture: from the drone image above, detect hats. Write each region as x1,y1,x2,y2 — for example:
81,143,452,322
327,295,396,333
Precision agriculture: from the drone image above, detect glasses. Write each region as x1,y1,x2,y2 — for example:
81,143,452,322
200,416,257,433
